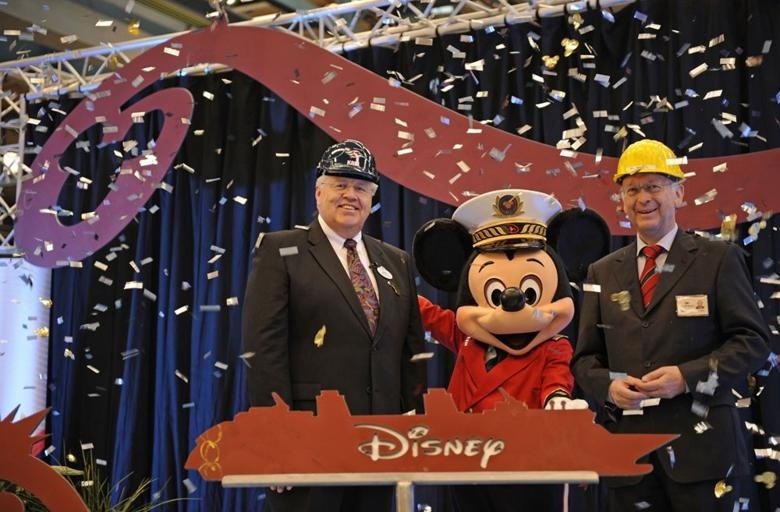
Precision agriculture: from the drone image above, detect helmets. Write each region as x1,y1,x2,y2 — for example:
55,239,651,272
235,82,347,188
612,137,686,186
317,137,380,183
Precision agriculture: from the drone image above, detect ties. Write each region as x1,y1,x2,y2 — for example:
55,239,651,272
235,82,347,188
343,238,380,338
637,244,662,309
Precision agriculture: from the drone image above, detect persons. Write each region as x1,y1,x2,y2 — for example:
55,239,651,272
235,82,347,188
239,136,429,511
695,299,705,309
566,137,769,512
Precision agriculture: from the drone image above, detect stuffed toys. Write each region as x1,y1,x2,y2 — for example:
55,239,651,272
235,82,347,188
408,183,615,512
